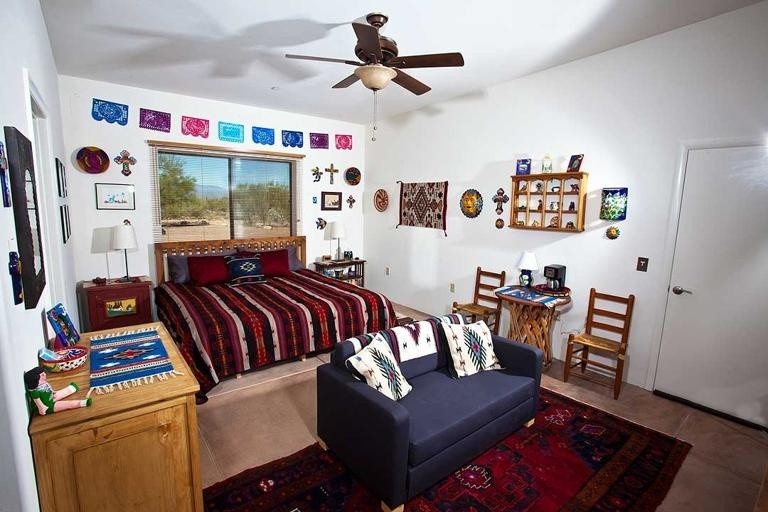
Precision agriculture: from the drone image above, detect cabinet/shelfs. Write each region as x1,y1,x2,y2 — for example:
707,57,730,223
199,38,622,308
511,171,589,233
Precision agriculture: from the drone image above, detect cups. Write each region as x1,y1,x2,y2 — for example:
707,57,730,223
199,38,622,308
335,269,344,278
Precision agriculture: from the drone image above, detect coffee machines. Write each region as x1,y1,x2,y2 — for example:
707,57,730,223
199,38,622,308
543,264,566,292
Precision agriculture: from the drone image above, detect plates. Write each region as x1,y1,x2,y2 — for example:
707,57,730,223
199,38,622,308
534,285,572,297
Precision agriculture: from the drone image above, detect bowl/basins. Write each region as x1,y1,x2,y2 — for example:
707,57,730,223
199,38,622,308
550,201,563,210
92,278,106,283
552,187,560,192
41,346,89,373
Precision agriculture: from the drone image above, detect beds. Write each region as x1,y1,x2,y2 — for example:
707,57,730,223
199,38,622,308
152,236,399,404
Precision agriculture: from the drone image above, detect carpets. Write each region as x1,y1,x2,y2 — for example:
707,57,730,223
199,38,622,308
395,312,416,326
203,440,384,512
403,385,692,512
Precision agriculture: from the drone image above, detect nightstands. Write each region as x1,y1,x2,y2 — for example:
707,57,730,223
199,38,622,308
314,259,367,288
76,275,153,332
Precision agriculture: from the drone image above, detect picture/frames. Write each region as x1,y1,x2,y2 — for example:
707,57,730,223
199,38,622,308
4,126,46,309
321,191,342,211
95,183,135,210
56,157,71,243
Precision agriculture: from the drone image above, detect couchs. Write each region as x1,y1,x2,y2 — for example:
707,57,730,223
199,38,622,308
316,312,544,510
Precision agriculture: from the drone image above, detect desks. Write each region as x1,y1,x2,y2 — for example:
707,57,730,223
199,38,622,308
27,321,204,512
494,285,573,373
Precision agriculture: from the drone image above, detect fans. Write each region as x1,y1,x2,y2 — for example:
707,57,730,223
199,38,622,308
284,12,464,95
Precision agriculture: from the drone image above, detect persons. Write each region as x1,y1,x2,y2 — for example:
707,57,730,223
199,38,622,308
25,366,91,416
461,189,480,218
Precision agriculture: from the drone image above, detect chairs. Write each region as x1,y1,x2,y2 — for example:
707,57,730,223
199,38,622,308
451,266,505,336
564,288,634,400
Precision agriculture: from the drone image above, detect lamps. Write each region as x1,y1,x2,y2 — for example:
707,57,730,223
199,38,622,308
354,63,398,142
599,187,627,221
112,219,138,282
331,221,345,262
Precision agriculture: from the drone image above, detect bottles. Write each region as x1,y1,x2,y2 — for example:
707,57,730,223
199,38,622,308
537,183,541,192
568,202,574,211
345,251,353,260
541,155,553,173
519,270,532,287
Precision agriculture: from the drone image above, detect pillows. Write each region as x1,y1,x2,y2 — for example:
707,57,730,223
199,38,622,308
334,312,506,402
167,246,302,287
